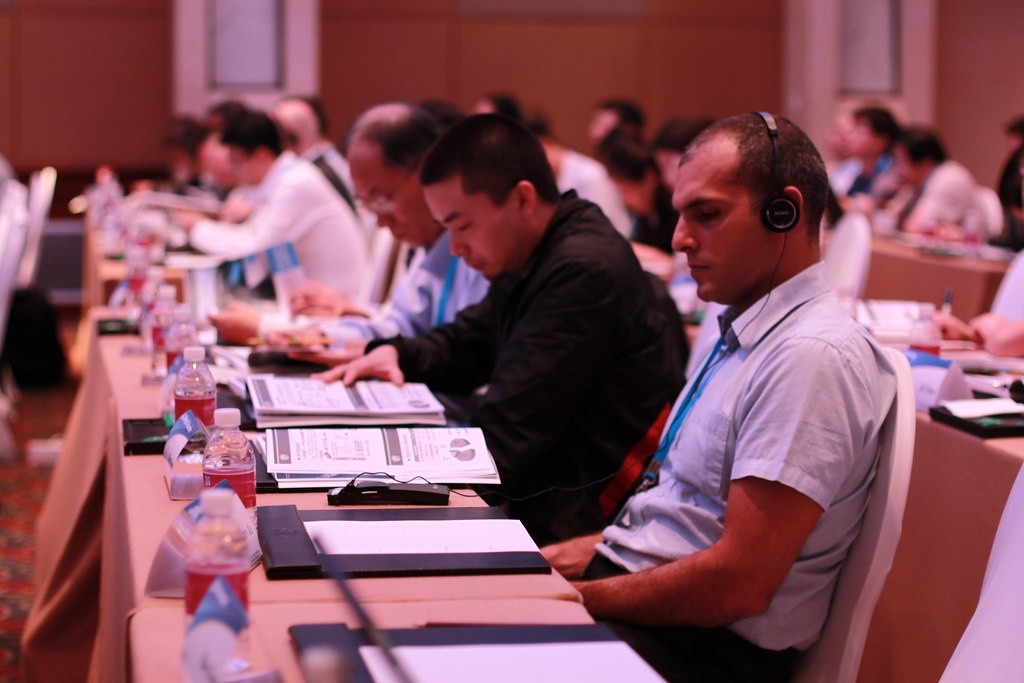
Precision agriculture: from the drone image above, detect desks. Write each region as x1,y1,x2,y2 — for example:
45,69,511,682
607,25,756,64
81,192,1024,683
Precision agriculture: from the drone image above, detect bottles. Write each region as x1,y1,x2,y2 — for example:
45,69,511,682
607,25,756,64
139,265,164,312
165,305,197,374
152,285,179,378
175,346,218,455
202,408,258,534
128,238,150,280
184,485,251,656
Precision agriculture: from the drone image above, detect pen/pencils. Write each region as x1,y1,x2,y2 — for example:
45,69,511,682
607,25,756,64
940,291,954,318
414,621,570,628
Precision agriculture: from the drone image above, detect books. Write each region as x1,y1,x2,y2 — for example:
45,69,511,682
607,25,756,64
227,375,448,428
255,425,501,488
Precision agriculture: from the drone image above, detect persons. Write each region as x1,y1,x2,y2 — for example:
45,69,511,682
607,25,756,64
134,86,1024,683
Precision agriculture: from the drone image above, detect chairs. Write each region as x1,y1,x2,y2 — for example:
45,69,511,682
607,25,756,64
790,347,916,683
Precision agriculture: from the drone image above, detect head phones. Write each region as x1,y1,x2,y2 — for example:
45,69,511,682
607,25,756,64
753,109,800,235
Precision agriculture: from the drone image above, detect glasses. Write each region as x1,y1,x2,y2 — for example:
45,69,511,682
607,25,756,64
352,158,420,222
